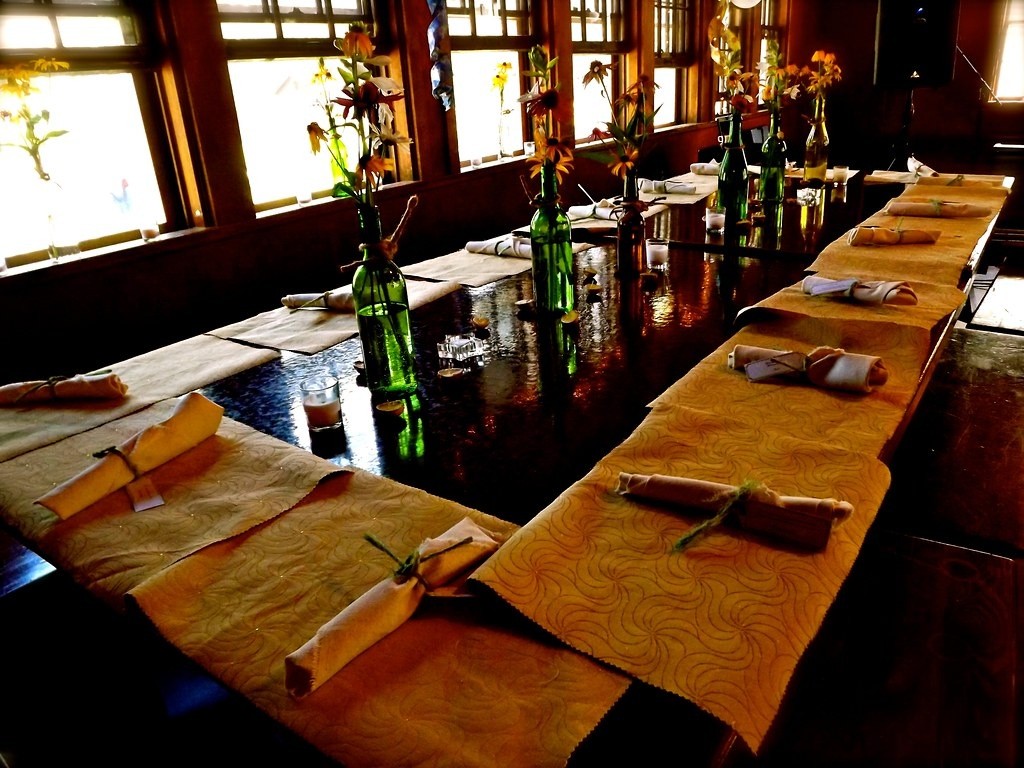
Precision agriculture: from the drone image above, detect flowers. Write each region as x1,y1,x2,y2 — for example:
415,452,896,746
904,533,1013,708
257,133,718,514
582,59,663,264
714,38,841,200
495,44,576,307
305,20,421,386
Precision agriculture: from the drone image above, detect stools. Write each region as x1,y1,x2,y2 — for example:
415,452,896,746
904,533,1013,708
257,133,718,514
720,249,1024,767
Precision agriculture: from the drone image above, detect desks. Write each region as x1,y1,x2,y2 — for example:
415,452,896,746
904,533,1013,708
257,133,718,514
0,164,1016,768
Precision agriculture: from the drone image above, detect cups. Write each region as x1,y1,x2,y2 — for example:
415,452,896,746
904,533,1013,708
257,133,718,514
471,151,482,168
833,167,848,187
370,171,382,191
299,376,342,432
138,216,160,240
0,256,8,274
646,239,668,270
524,142,535,157
706,208,725,235
295,183,312,205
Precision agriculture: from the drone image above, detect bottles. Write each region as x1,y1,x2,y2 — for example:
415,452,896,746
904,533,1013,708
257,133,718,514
760,111,789,202
528,165,575,316
350,206,418,396
715,115,750,235
326,117,352,197
615,168,645,281
802,99,831,188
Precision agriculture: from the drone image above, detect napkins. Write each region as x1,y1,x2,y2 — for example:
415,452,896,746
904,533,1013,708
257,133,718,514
284,515,503,702
690,163,720,174
883,200,992,218
615,472,855,547
907,155,992,189
280,293,355,311
33,391,224,520
848,227,940,244
0,369,130,404
465,238,533,259
727,344,888,393
565,198,625,221
637,178,696,196
801,275,918,306
